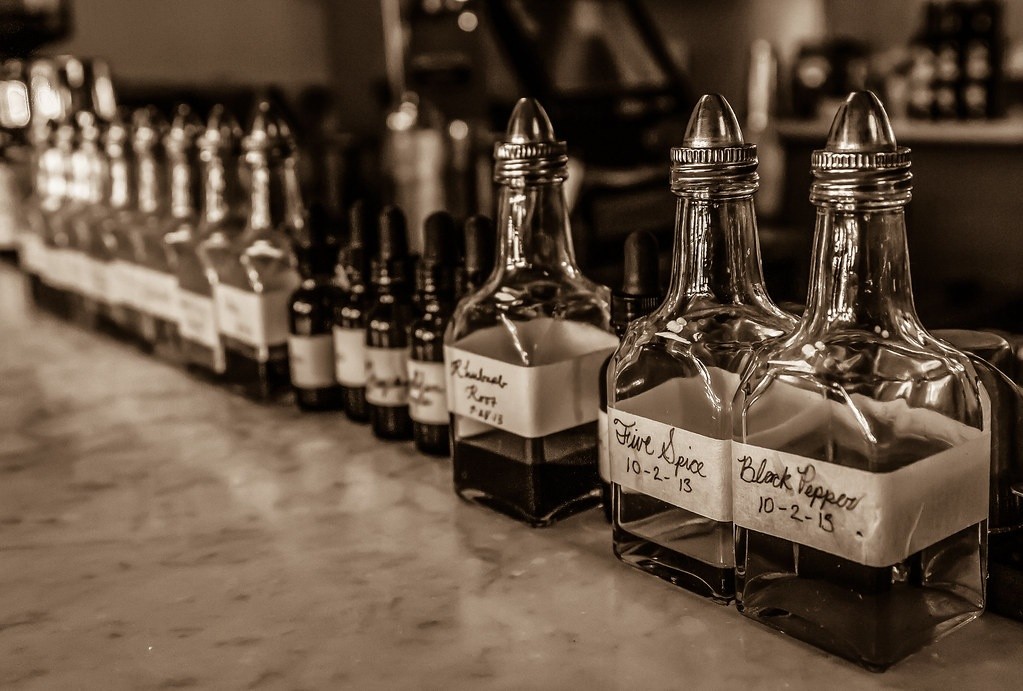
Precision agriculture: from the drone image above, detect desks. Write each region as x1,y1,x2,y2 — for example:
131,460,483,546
0,263,1023,691
771,123,1023,331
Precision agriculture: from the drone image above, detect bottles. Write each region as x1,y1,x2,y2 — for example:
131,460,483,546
729,88,994,673
598,91,803,606
0,56,304,406
443,97,620,528
287,201,496,458
